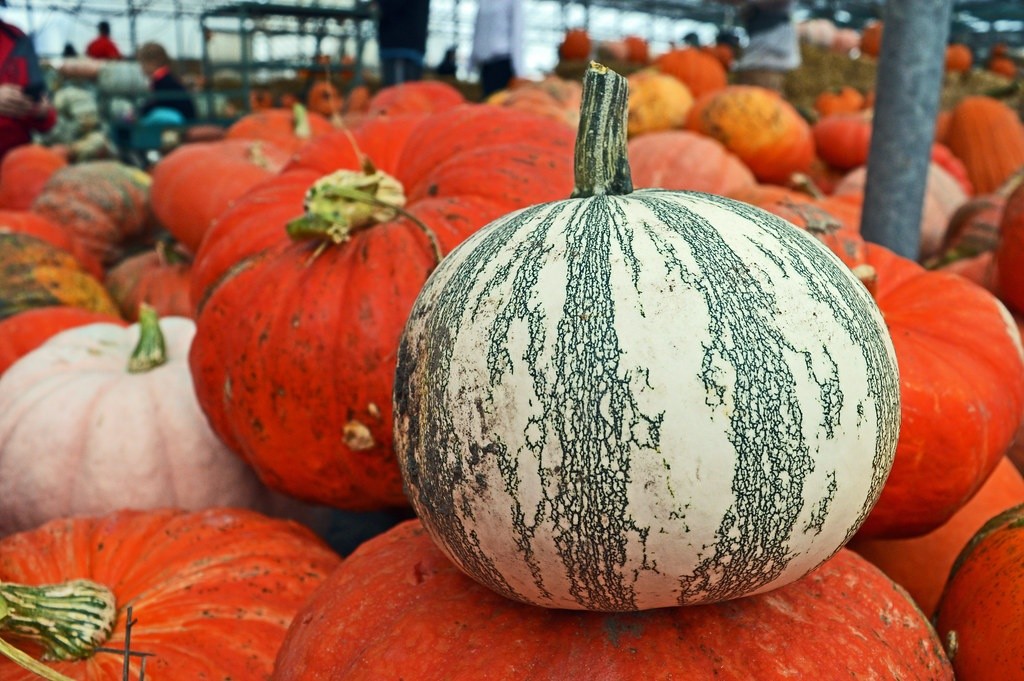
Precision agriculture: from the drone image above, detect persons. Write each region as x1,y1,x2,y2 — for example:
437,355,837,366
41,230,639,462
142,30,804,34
0,17,57,157
61,20,123,61
139,43,198,126
464,0,522,101
681,0,803,96
373,0,431,89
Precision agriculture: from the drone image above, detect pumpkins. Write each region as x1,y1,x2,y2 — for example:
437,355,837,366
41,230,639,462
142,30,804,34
0,17,1024,681
